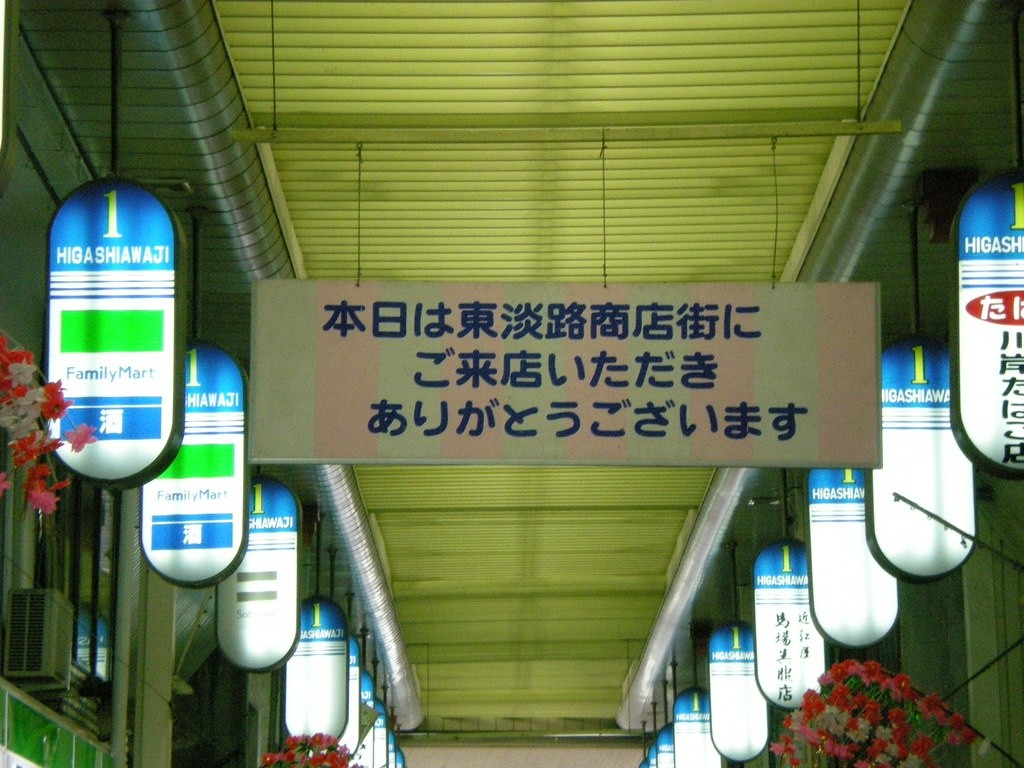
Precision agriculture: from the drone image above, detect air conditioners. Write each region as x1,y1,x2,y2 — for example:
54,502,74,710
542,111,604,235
2,588,76,694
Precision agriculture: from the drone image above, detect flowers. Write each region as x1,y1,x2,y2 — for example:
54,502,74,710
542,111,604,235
260,733,368,768
0,334,98,541
769,658,980,768
